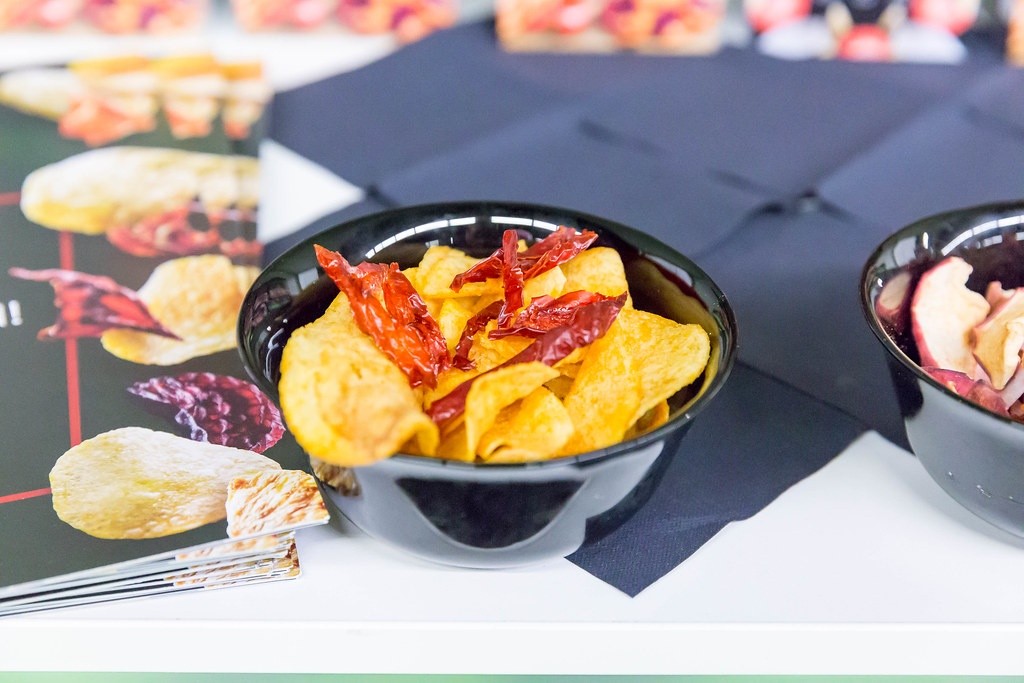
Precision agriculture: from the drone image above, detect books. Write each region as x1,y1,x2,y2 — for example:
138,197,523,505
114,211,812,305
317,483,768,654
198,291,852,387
1,51,331,613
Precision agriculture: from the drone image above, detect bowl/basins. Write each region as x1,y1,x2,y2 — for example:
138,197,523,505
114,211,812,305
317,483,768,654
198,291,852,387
234,197,738,571
857,200,1024,541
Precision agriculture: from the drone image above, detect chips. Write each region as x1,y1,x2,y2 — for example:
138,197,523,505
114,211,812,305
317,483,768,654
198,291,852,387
0,61,326,541
279,236,708,467
